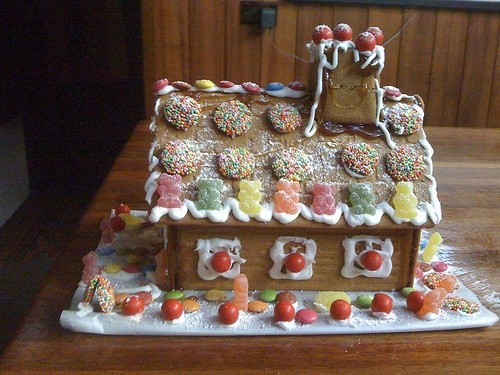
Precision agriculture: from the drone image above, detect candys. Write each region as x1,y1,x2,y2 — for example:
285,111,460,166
312,23,385,52
76,203,478,326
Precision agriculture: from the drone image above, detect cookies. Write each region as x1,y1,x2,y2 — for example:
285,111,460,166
142,37,444,293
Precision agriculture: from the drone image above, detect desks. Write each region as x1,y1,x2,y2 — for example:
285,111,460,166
0,109,500,375
